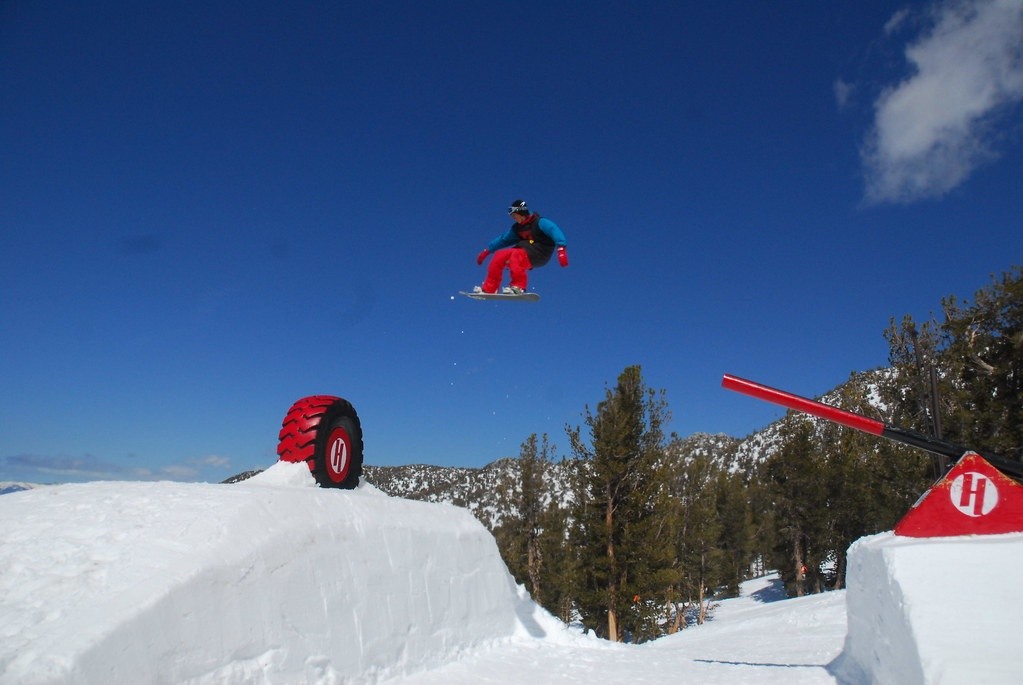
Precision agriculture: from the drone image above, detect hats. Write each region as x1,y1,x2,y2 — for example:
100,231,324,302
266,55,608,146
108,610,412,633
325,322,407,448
512,200,529,213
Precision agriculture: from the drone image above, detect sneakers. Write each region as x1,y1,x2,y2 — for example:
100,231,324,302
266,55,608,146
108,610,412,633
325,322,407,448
503,286,524,294
474,285,497,295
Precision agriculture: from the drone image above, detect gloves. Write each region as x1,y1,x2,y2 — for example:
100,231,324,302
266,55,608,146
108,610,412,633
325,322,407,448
557,246,568,266
477,249,490,265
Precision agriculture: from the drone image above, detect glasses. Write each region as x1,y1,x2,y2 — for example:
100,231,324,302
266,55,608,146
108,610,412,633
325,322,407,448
506,207,527,215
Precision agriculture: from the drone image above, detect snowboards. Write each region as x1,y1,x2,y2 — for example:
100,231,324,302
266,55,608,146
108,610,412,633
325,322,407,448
458,290,540,303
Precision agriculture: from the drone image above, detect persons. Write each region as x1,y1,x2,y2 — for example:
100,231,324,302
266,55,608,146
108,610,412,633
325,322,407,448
801,561,809,595
473,200,569,293
